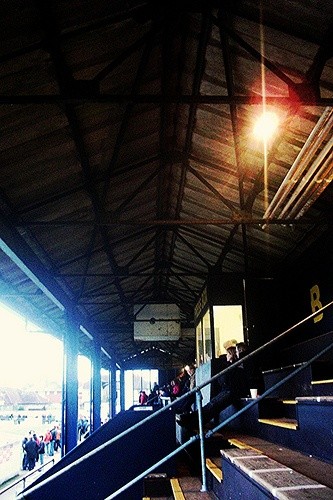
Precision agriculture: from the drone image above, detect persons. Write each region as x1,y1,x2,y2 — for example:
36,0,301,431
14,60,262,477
121,339,247,407
7,412,100,471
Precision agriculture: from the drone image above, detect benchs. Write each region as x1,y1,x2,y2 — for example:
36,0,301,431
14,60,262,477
262,362,333,461
220,448,333,500
